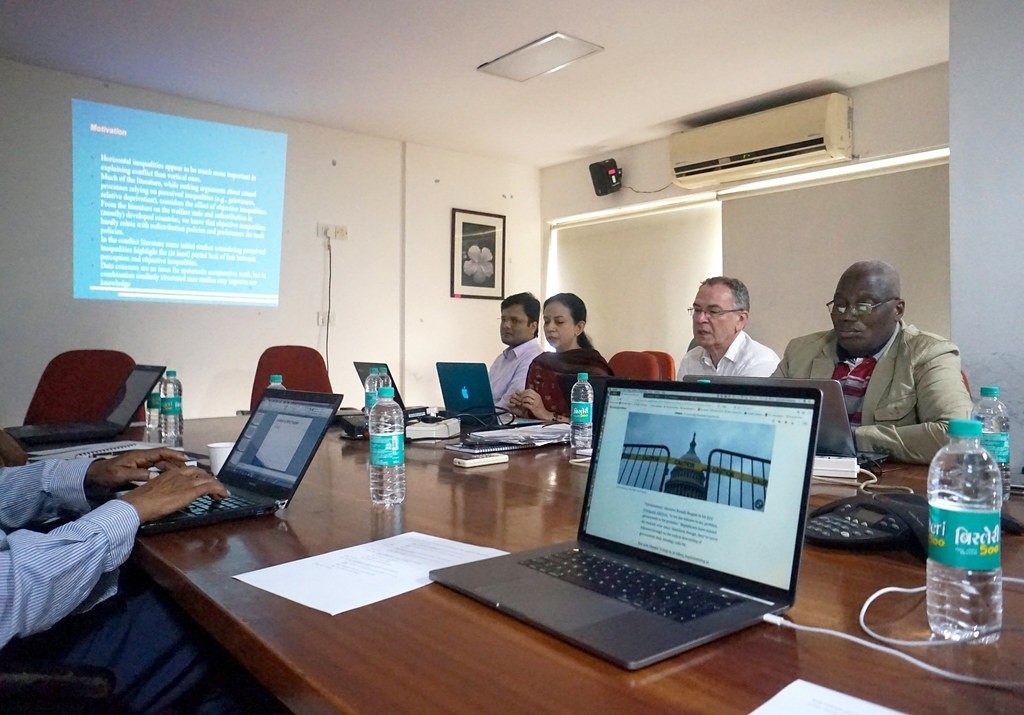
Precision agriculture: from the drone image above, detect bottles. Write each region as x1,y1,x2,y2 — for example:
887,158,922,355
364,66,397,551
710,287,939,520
697,379,711,382
267,374,286,389
159,370,183,438
971,387,1010,503
369,388,405,505
379,368,392,388
365,368,384,418
570,373,593,449
146,377,166,431
925,418,1003,645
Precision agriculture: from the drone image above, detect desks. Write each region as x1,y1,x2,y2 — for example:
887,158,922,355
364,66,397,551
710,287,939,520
20,409,1024,715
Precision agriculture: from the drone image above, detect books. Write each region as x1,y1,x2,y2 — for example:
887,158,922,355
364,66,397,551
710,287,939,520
445,444,534,453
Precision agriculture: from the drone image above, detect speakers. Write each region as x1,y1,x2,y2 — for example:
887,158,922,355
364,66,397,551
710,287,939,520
589,158,621,197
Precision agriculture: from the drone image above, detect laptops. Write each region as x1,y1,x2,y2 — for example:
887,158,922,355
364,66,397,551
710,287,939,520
555,375,615,444
2,365,168,446
436,362,546,426
430,377,823,668
683,374,890,468
100,389,344,536
353,361,430,414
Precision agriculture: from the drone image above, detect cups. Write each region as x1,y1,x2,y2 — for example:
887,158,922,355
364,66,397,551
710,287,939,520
208,442,235,479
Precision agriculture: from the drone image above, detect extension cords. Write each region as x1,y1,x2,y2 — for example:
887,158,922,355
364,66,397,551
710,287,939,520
811,452,860,479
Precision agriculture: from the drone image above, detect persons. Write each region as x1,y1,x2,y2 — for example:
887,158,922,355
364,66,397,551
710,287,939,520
507,292,615,425
677,276,780,382
488,293,544,415
0,448,283,715
770,259,974,465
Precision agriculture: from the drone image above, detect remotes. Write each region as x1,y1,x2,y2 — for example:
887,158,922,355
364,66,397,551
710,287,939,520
453,454,509,468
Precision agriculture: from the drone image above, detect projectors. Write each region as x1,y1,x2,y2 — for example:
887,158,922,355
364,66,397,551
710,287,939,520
340,414,370,437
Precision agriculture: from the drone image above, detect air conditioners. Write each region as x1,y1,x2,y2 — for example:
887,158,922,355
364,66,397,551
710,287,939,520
668,90,858,189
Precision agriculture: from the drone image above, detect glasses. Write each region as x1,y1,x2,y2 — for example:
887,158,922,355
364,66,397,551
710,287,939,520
688,307,744,317
826,297,901,316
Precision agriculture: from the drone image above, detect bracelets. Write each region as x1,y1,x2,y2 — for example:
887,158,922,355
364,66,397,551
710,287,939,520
552,413,558,421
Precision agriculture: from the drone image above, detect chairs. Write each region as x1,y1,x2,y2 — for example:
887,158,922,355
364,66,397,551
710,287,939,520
24,349,135,424
251,346,335,415
608,351,675,384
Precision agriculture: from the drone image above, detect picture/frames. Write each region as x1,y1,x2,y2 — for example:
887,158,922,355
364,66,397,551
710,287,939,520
450,208,506,300
